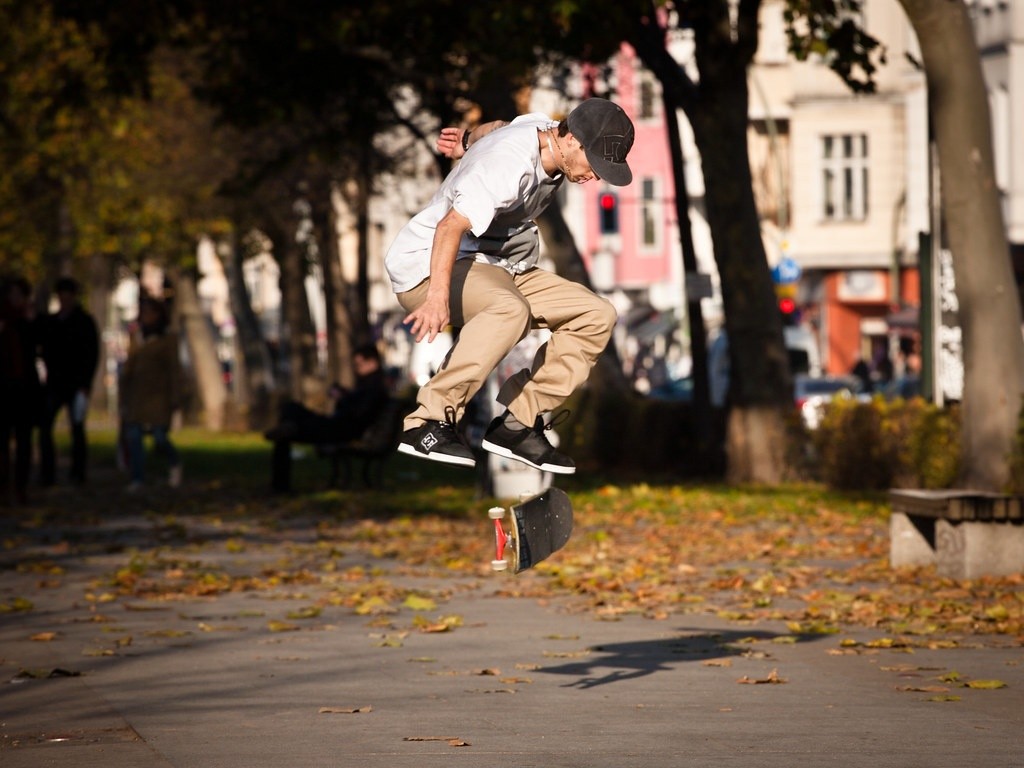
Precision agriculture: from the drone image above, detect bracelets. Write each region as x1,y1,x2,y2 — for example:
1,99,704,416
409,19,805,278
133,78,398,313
462,128,471,152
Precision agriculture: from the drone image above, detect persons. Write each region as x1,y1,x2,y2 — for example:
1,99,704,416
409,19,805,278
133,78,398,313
385,100,634,475
252,345,390,494
849,352,923,395
117,298,188,498
1,273,98,503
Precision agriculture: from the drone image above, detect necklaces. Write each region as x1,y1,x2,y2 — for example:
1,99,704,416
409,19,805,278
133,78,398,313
546,118,572,178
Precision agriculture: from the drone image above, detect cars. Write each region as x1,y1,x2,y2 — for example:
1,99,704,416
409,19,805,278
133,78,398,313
647,366,924,433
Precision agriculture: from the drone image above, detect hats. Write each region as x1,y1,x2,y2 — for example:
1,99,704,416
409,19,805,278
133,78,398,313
566,97,635,186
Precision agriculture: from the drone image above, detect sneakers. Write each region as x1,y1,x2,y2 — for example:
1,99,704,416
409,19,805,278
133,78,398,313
397,419,476,468
481,413,577,475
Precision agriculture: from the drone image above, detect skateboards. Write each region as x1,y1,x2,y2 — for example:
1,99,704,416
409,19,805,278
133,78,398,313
488,486,572,574
